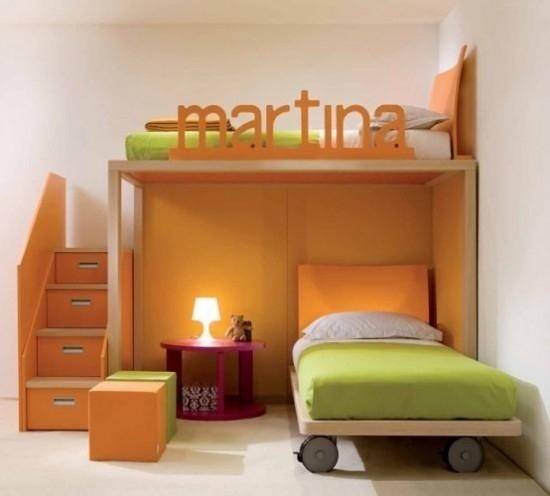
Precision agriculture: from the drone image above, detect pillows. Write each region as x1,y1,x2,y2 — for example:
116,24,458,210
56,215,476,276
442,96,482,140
302,311,445,342
364,102,451,134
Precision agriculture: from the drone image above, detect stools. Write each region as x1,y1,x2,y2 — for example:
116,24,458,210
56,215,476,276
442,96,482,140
86,380,167,463
106,370,178,446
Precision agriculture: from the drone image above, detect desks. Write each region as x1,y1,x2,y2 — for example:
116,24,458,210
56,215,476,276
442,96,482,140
158,336,266,422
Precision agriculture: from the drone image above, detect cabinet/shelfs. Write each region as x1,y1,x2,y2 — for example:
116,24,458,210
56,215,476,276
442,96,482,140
20,245,135,432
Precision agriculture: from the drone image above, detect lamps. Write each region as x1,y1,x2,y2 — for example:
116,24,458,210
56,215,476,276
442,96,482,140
191,294,224,345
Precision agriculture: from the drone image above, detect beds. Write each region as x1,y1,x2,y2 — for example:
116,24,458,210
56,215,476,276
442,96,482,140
287,261,523,480
108,39,478,375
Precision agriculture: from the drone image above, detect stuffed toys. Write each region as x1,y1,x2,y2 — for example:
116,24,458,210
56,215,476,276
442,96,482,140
224,312,253,342
